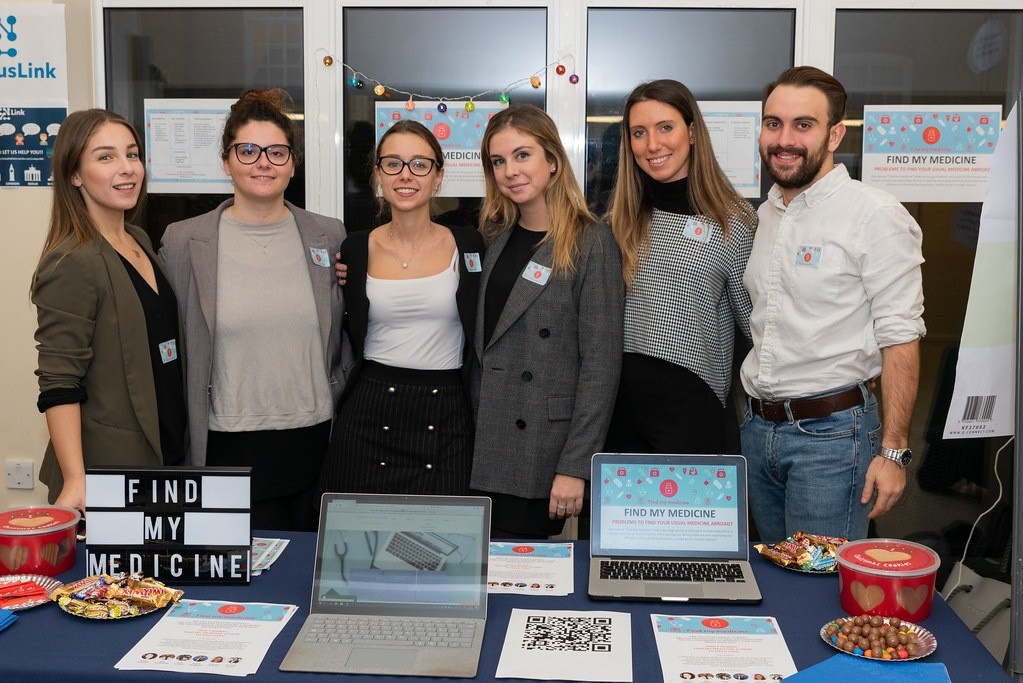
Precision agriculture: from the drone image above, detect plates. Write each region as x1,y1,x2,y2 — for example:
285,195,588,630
772,560,839,573
0,574,64,611
820,617,937,661
59,602,159,620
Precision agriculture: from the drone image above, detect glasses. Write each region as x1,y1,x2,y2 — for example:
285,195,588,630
377,157,442,177
225,142,297,167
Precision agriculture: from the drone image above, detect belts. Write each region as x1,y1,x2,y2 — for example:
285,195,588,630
745,381,872,424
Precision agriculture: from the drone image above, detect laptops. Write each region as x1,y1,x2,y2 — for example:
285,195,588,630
279,493,492,678
373,532,459,571
588,453,763,604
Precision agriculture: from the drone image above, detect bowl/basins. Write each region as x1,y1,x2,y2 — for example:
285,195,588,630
836,538,941,623
0,505,81,577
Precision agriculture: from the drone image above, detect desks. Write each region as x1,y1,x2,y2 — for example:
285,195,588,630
0,527,1012,683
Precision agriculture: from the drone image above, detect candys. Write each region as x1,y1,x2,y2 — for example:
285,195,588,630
50,573,185,617
754,531,850,571
827,614,921,659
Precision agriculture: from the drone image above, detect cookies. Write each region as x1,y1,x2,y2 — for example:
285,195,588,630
0,537,70,571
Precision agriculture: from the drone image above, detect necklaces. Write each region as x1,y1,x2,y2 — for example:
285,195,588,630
232,205,287,248
389,222,430,269
102,232,140,258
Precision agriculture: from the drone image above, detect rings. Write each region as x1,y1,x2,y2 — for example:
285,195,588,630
557,504,567,509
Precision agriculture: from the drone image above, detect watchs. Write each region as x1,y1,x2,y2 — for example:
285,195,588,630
878,444,913,468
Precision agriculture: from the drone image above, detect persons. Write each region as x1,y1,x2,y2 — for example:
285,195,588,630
330,120,478,495
157,90,348,534
740,66,925,541
488,582,554,589
31,108,191,518
576,79,877,538
681,673,782,680
142,653,239,663
335,104,624,542
